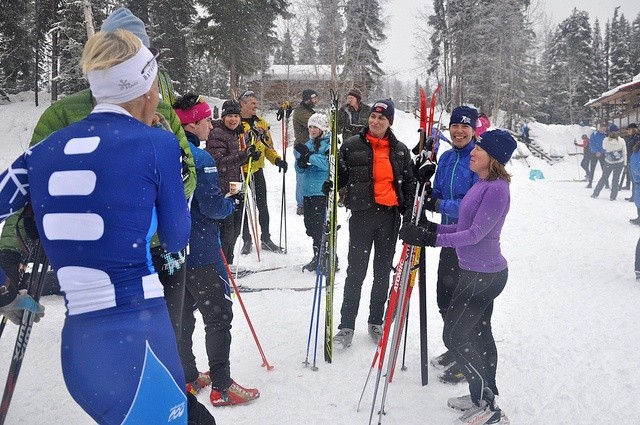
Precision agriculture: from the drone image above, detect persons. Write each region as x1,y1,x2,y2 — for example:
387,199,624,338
574,134,591,177
622,122,640,202
591,124,628,201
398,129,517,425
235,91,288,256
293,111,340,275
171,91,261,408
0,29,192,425
336,87,370,143
425,126,451,162
320,97,415,350
627,136,640,281
206,100,261,277
586,123,610,189
411,106,481,385
292,89,320,215
0,6,198,347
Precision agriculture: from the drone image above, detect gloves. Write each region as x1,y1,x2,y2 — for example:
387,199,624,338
0,248,30,293
276,159,287,173
296,156,307,169
246,145,255,156
253,151,261,160
228,190,246,208
321,180,333,196
1,293,45,325
421,196,436,211
294,143,312,161
400,222,436,247
403,209,437,232
154,112,174,132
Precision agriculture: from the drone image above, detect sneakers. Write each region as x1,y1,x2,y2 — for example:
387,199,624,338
210,380,259,407
225,264,248,274
430,349,454,366
453,400,501,424
369,322,383,344
241,239,253,254
185,371,210,396
302,258,318,273
448,393,475,411
435,364,467,384
260,234,284,253
332,328,353,349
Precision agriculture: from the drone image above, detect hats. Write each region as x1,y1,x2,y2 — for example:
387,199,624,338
450,107,477,130
476,129,517,166
308,110,329,133
99,7,150,47
348,88,361,99
368,98,394,125
302,89,319,100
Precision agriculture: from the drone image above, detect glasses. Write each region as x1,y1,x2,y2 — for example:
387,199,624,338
242,91,254,98
142,48,160,74
193,95,205,105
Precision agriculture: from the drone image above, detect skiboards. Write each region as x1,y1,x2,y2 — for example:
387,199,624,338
324,87,340,362
229,88,261,260
276,101,293,254
369,98,456,424
417,84,443,384
226,264,337,294
